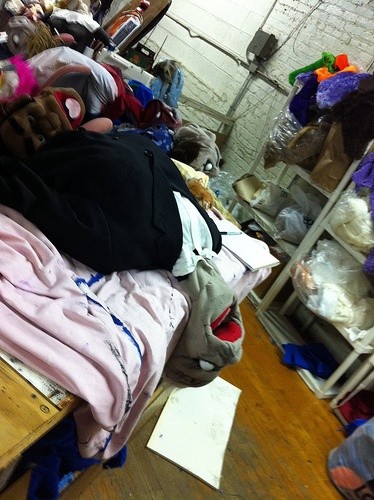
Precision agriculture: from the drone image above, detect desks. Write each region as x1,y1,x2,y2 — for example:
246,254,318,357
0,335,79,488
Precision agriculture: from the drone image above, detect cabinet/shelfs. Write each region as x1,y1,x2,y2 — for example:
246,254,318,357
226,74,374,426
